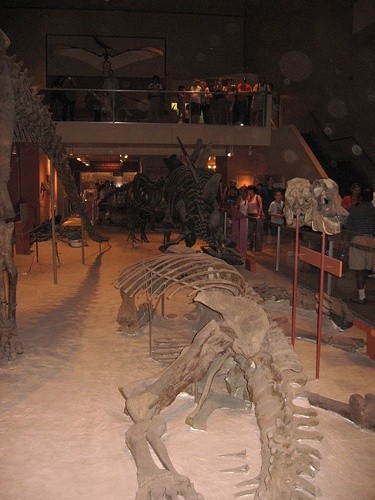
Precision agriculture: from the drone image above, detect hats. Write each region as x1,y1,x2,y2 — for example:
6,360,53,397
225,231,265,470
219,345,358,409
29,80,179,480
246,185,257,190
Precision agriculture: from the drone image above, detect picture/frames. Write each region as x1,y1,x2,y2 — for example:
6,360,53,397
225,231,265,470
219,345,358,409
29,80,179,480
45,34,166,80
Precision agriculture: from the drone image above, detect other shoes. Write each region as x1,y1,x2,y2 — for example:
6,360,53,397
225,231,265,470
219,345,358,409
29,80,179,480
350,297,367,305
247,246,253,250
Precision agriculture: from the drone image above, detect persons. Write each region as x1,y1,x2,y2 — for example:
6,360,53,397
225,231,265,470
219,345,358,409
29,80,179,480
237,77,252,124
342,183,375,305
189,79,203,125
249,77,272,124
238,187,249,249
343,188,375,304
102,69,121,121
342,183,362,210
219,177,286,252
178,77,280,125
247,184,265,252
268,189,285,226
49,75,75,121
147,75,162,122
